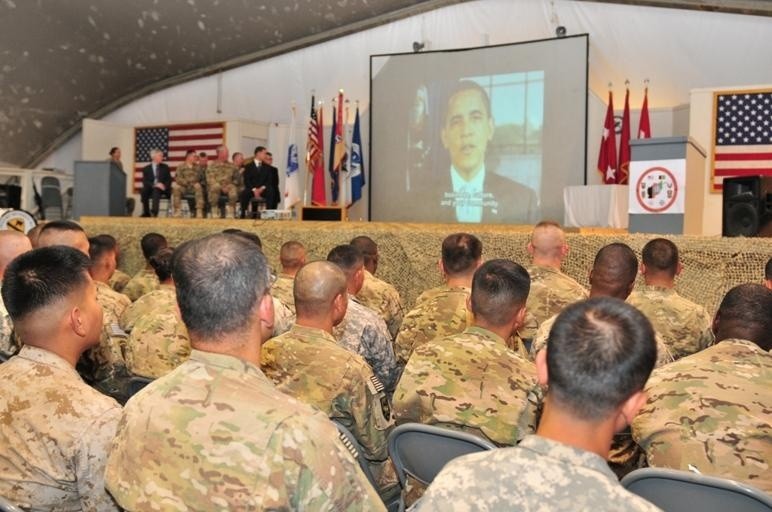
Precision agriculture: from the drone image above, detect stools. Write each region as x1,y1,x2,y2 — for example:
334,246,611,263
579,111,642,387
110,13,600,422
148,192,265,219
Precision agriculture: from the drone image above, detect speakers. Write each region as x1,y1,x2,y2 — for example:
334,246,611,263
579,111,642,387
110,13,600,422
721,173,772,238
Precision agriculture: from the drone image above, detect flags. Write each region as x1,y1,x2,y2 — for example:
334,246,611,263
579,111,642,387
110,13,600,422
708,85,772,196
618,87,632,186
284,89,365,213
133,120,227,196
638,90,653,139
598,88,616,183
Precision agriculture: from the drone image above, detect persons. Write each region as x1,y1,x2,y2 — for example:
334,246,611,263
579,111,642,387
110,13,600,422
630,282,772,496
407,294,666,512
390,259,549,446
405,82,435,166
260,261,398,493
0,245,124,512
407,82,540,222
103,231,388,512
107,145,282,220
0,220,715,404
532,241,649,481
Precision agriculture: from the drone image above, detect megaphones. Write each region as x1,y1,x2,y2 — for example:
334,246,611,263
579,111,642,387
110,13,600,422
412,41,426,51
556,26,566,38
